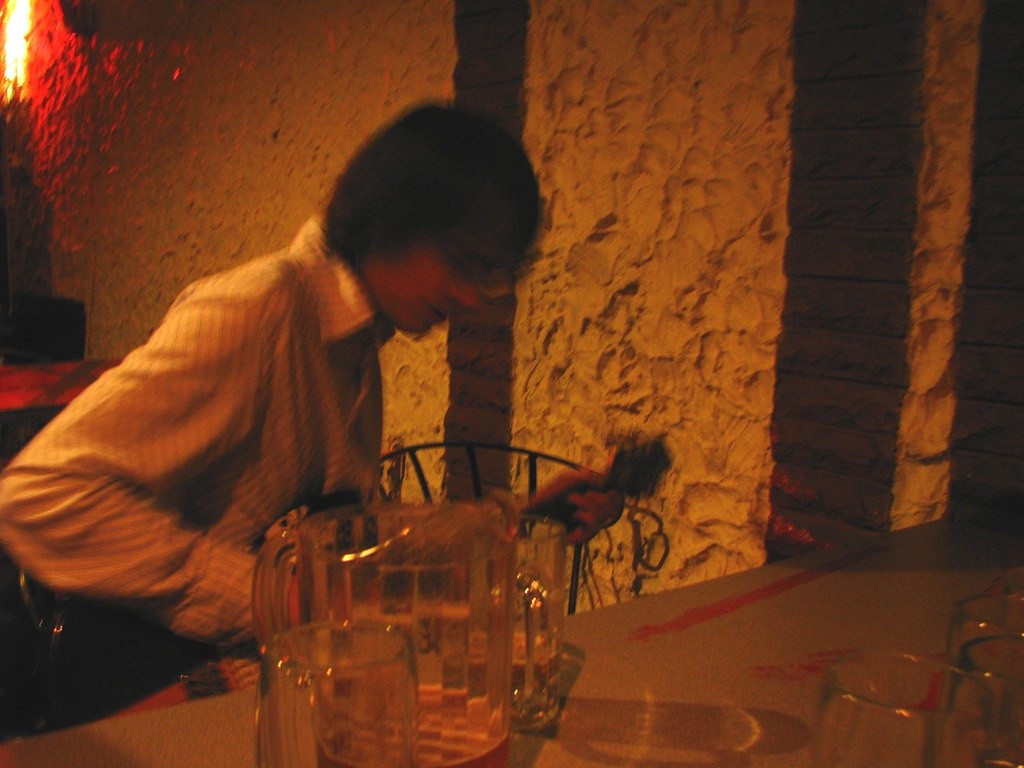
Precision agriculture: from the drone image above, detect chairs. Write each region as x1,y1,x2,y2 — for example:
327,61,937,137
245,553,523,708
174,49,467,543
377,440,600,617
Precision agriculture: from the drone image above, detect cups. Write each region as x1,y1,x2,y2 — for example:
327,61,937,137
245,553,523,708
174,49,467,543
810,591,1024,768
251,497,567,767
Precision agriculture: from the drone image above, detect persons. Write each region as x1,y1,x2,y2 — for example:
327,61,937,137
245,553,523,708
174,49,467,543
0,100,626,647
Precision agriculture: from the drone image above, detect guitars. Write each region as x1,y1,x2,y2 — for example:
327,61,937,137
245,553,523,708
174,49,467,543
95,423,681,723
